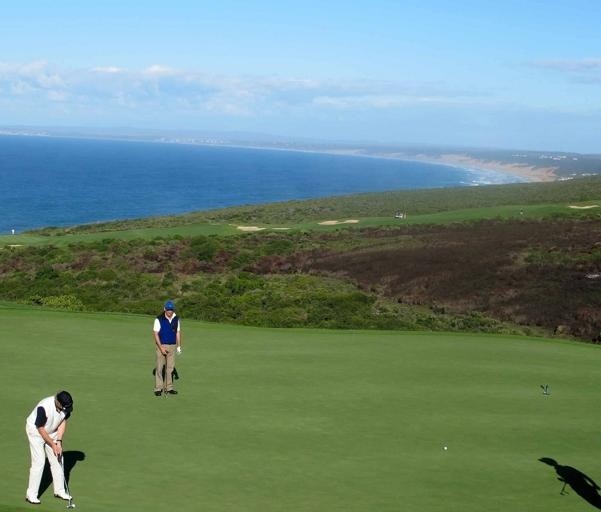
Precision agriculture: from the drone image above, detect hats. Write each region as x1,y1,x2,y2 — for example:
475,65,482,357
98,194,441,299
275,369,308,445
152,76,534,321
58,391,73,413
164,301,175,311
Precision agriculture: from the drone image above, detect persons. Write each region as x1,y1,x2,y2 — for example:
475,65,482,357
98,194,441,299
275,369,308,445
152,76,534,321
24,391,74,504
152,300,182,396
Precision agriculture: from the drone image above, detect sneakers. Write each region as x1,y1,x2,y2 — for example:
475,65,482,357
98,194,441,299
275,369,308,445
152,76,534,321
156,391,161,396
26,496,41,504
54,492,72,500
170,390,177,394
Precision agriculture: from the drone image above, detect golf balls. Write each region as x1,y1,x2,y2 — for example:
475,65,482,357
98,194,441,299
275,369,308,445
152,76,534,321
72,503,76,508
444,446,448,451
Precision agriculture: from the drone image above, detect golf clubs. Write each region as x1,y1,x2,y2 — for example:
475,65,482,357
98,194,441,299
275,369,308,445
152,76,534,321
57,454,72,509
164,352,169,394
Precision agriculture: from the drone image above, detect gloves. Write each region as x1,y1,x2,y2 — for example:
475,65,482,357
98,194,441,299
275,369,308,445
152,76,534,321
176,346,182,354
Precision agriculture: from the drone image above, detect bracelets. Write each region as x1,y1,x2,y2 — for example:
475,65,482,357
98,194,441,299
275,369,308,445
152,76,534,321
56,439,62,442
